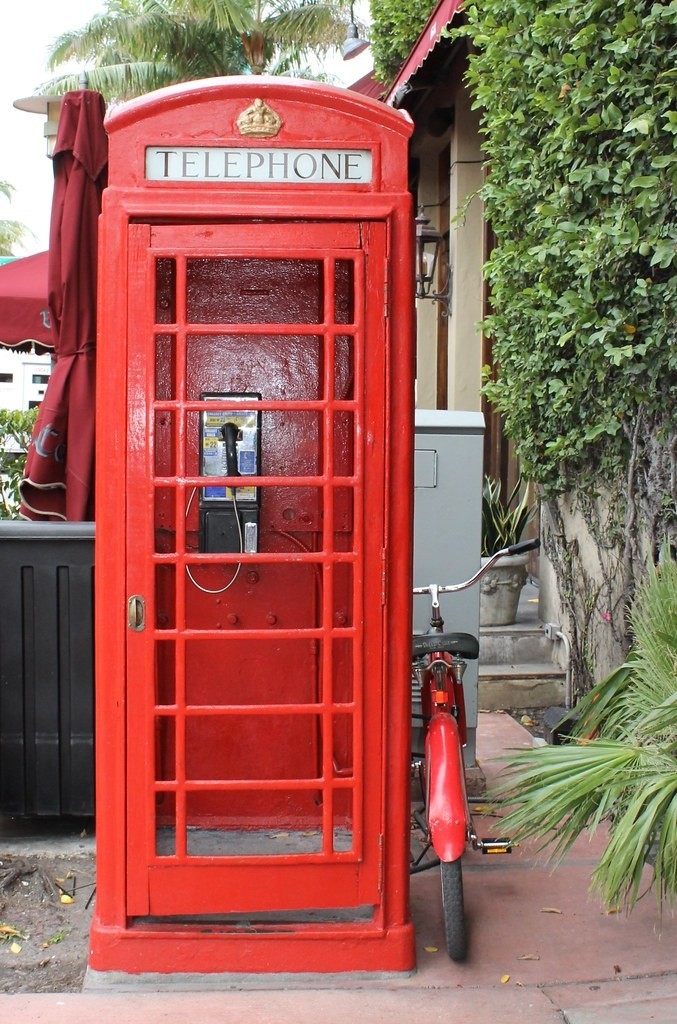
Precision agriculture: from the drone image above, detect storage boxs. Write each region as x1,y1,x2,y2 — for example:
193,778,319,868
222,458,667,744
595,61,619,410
543,707,582,746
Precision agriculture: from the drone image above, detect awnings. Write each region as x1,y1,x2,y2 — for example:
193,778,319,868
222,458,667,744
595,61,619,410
1,251,56,356
345,0,467,107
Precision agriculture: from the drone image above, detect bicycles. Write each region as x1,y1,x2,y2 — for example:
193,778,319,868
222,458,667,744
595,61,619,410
410,538,541,963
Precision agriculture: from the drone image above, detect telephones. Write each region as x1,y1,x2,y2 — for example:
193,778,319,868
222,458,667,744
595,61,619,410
201,392,260,566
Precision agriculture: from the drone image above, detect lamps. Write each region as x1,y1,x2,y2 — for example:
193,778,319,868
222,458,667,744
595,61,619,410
415,203,452,318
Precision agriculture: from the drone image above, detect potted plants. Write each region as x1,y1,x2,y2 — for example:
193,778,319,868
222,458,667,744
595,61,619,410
481,471,540,626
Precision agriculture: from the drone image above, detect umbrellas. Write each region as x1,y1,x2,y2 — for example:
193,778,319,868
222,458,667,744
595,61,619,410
17,91,108,522
